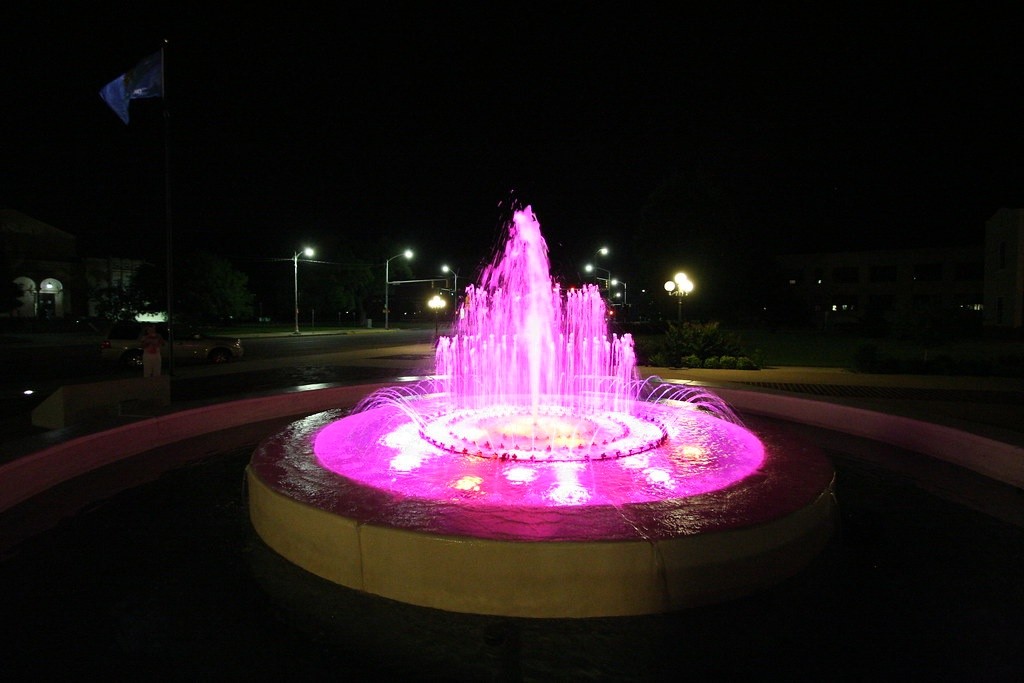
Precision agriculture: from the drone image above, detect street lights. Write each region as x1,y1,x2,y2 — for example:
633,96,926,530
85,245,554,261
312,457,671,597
384,249,411,330
665,273,694,364
585,264,612,311
291,246,316,333
593,247,610,286
441,264,459,320
610,277,628,305
427,294,448,339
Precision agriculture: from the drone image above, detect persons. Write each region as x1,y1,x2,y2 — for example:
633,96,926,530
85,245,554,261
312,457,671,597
142,326,166,377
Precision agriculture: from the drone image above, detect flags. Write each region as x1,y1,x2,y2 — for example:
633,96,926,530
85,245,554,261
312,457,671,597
98,50,162,125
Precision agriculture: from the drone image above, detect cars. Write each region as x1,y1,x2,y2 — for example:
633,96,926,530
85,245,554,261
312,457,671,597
100,322,246,372
